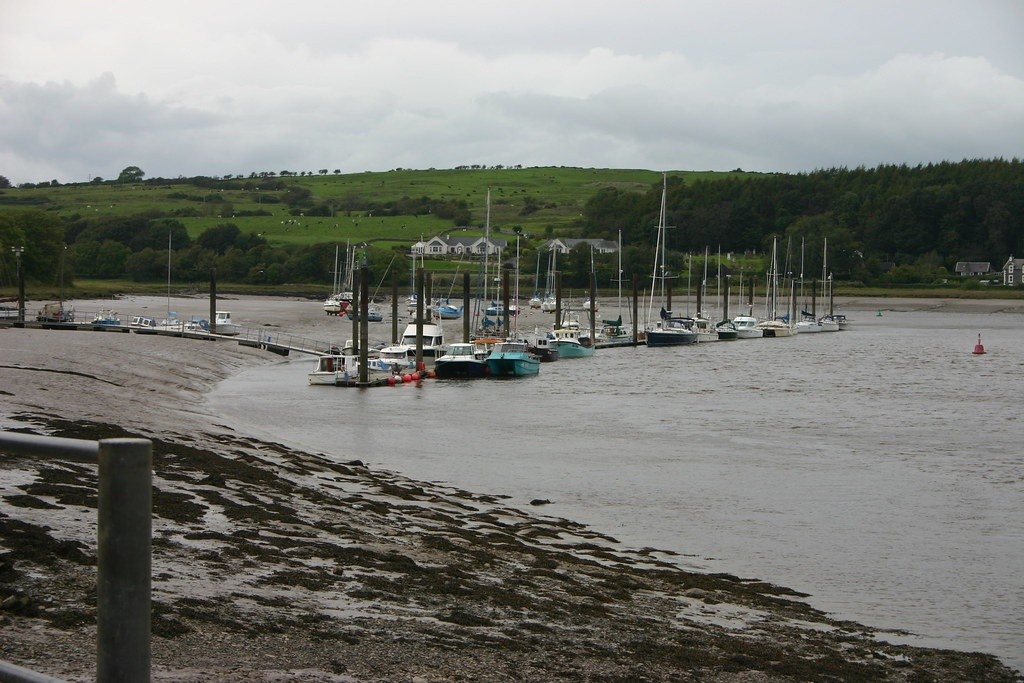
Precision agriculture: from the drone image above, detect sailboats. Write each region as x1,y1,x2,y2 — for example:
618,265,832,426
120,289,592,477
161,232,185,329
307,183,848,389
36,243,75,322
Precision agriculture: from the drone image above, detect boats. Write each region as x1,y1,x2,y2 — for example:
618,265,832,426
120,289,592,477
131,315,157,328
0,306,20,318
184,315,209,331
209,311,242,336
91,304,120,325
